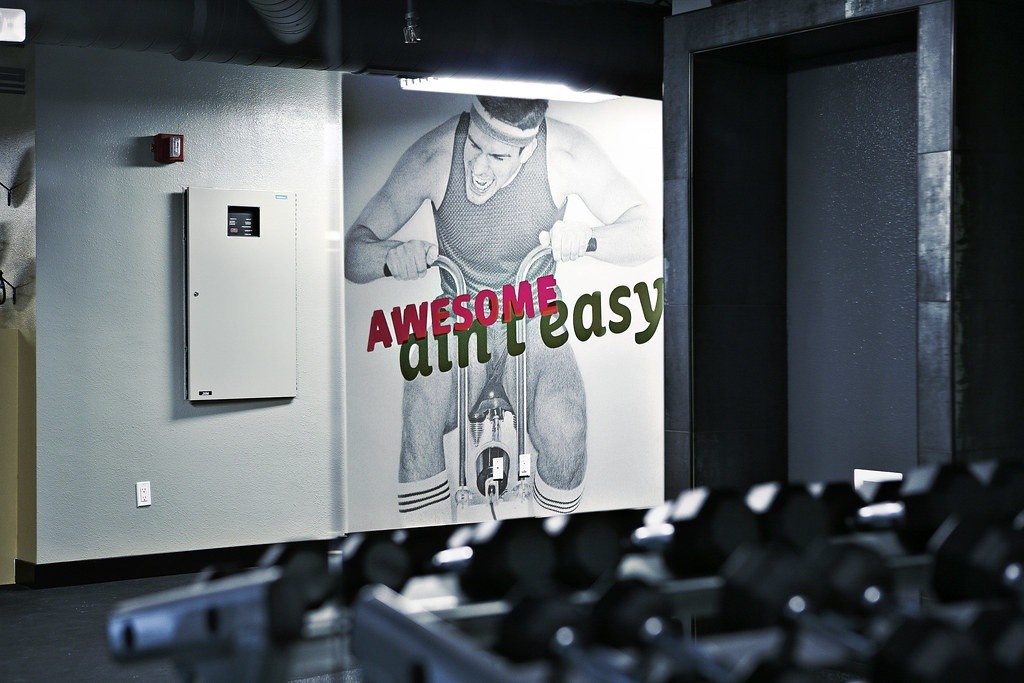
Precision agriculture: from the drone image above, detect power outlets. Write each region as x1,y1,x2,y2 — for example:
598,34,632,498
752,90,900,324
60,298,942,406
136,481,151,508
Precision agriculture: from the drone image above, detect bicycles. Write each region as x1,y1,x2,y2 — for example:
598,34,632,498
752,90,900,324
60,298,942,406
383,236,597,524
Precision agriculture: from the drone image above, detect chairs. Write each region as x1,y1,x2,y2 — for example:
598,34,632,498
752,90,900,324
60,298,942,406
104,457,1023,683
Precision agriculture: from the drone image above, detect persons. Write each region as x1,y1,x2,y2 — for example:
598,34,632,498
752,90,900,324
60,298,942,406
344,93,663,527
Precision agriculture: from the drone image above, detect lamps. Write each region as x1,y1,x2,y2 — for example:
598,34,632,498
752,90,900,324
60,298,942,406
403,0,422,45
0,7,27,46
397,75,622,103
